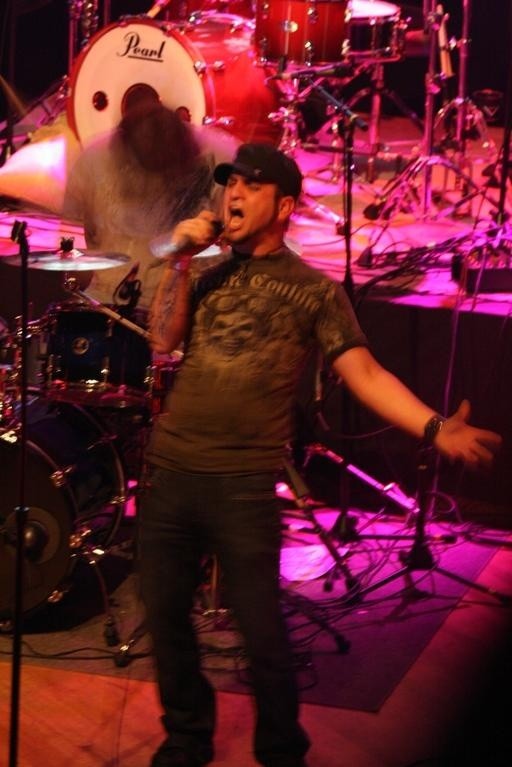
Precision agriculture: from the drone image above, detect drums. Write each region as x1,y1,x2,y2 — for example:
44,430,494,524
63,8,306,168
0,395,126,619
36,303,155,408
346,0,402,57
250,0,350,67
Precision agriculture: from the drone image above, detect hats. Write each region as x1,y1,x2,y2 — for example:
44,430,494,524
213,143,303,195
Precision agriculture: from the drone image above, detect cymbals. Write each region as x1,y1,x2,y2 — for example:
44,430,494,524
7,251,129,273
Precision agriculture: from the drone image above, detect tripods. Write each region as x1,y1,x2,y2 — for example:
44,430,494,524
326,255,512,608
280,126,457,593
276,461,366,597
111,553,351,666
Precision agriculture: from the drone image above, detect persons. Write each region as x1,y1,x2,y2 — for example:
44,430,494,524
129,144,500,766
62,81,226,320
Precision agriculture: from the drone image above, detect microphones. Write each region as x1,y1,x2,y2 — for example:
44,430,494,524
166,221,224,261
119,265,138,301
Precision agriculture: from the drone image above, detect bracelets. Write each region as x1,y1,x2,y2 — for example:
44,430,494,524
422,413,446,447
